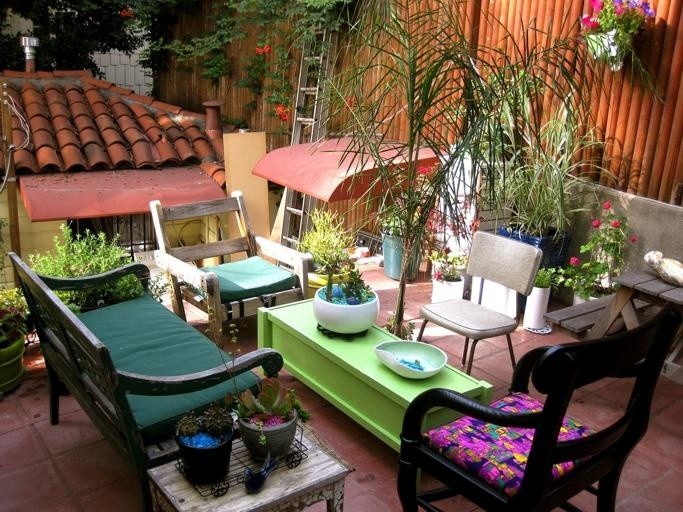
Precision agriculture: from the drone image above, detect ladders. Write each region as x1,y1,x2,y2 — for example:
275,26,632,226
277,27,338,276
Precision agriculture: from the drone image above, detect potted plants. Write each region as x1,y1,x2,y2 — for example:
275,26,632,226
175,405,233,484
231,373,299,460
297,205,379,334
0,286,29,395
304,1,659,334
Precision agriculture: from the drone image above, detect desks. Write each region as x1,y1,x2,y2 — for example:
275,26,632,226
254,295,496,493
550,255,683,366
144,420,357,510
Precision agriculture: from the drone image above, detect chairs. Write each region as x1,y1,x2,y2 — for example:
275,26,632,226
6,249,284,509
417,229,545,378
395,299,683,510
146,191,312,353
